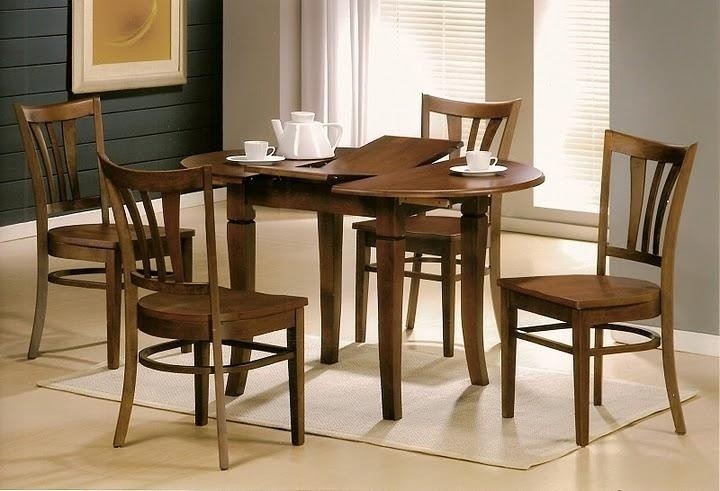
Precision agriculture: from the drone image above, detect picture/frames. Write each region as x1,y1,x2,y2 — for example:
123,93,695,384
66,0,189,96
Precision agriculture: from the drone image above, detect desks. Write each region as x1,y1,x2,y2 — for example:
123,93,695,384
180,146,541,421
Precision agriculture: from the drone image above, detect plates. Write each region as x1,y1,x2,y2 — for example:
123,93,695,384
225,156,285,166
450,166,508,177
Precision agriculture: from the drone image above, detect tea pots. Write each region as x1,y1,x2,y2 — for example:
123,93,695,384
271,111,343,160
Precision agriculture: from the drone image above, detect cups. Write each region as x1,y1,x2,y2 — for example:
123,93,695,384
466,151,497,170
244,141,275,159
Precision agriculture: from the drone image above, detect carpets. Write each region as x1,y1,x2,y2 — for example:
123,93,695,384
36,333,700,471
